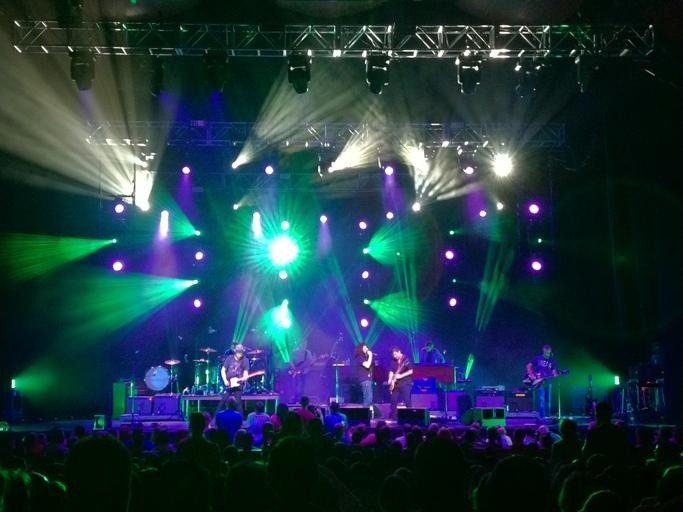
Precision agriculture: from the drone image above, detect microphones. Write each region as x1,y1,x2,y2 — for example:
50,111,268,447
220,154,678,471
366,351,378,356
422,346,429,351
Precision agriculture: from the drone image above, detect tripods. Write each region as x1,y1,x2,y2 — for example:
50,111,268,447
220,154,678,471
168,374,186,421
243,354,273,396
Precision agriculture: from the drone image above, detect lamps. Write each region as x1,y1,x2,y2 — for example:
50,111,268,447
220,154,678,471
67,49,599,97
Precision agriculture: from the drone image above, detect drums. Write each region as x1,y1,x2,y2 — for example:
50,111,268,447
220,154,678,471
147,367,171,391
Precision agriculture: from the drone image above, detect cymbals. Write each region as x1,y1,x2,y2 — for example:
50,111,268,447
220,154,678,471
165,360,179,365
193,359,210,364
248,348,262,355
200,348,219,354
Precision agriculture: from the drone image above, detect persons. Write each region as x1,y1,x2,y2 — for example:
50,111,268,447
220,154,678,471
0,401,683,512
223,341,239,357
352,342,374,407
642,350,663,381
288,338,314,407
387,345,414,419
207,344,248,428
526,344,558,417
418,340,444,365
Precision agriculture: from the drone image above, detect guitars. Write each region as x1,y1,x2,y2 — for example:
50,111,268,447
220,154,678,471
288,354,329,377
524,368,569,394
388,357,409,393
223,371,266,390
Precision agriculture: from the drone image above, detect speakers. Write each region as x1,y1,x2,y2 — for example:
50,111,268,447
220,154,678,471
127,395,154,416
460,406,506,427
442,388,467,410
120,413,142,425
152,392,182,416
410,376,439,411
475,390,507,407
339,407,371,427
396,406,431,427
111,382,127,419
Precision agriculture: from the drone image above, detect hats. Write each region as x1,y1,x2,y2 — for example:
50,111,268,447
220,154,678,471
232,344,246,354
